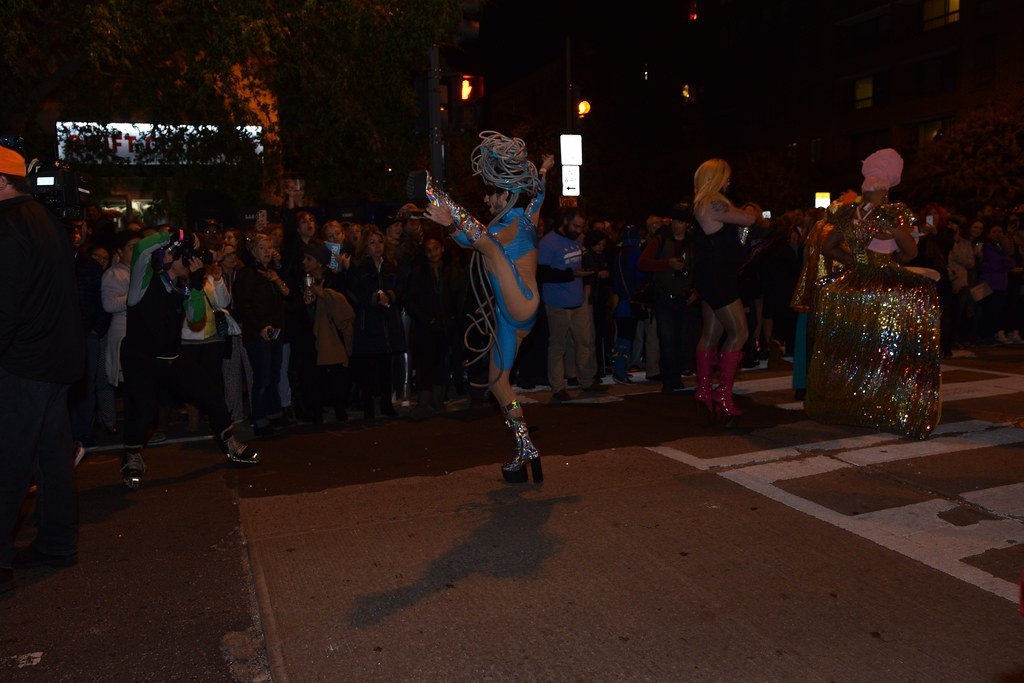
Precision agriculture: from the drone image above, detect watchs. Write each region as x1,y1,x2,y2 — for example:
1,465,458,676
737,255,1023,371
446,224,456,233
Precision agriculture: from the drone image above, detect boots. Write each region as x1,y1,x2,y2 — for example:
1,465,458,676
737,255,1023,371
405,170,489,244
222,436,262,463
121,444,146,488
712,352,745,419
694,349,716,416
501,416,544,484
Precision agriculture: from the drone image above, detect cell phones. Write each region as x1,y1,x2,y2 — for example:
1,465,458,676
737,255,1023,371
267,328,281,339
926,216,933,225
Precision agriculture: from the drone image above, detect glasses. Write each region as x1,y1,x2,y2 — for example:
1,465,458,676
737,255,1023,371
92,252,110,264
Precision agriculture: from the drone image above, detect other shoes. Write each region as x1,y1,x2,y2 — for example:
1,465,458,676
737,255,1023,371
583,381,609,392
675,374,685,389
662,380,673,396
995,333,1013,344
0,563,17,591
553,390,581,400
1012,334,1024,344
379,399,398,417
11,540,79,568
629,296,650,320
646,372,662,381
612,373,634,385
148,431,167,442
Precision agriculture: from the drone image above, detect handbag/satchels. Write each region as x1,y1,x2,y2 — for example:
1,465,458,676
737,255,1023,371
969,281,992,302
212,303,242,336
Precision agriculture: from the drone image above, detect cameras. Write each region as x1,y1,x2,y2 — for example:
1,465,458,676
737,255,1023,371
172,237,214,266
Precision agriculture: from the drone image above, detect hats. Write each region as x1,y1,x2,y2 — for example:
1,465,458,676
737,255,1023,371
0,146,27,177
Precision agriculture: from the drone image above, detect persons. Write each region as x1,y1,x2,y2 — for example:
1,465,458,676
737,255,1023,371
424,154,556,484
686,160,764,415
801,183,942,436
906,203,1024,358
510,189,867,403
26,200,496,488
0,144,83,580
